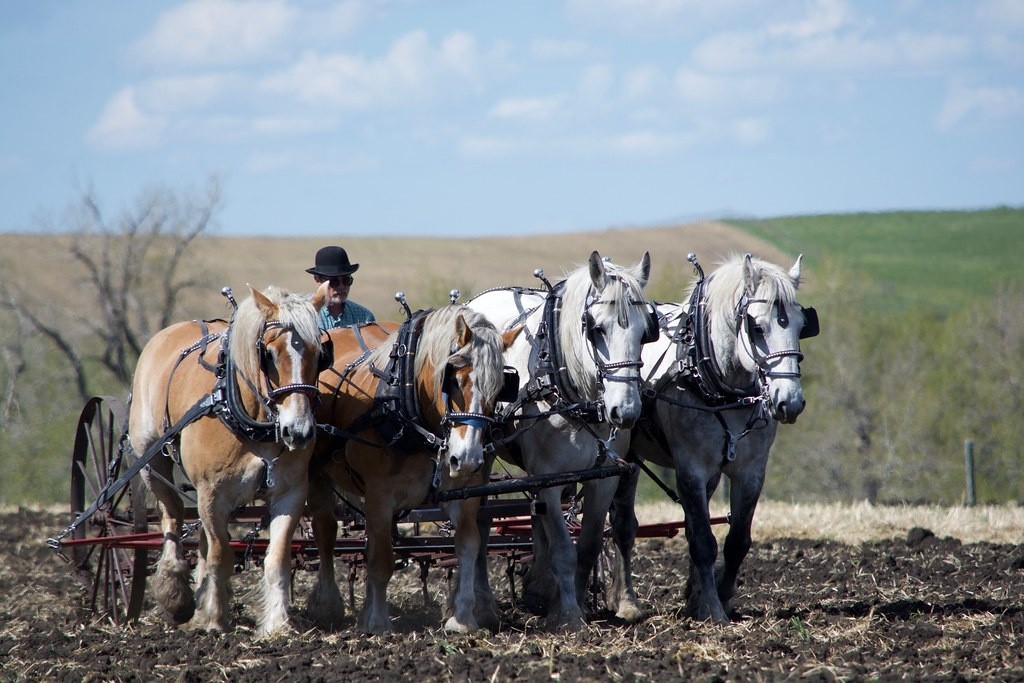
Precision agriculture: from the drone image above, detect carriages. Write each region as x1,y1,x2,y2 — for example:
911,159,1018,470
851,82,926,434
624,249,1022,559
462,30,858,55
45,244,820,646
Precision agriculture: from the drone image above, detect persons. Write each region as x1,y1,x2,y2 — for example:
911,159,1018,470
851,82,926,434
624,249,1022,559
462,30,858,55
305,246,375,331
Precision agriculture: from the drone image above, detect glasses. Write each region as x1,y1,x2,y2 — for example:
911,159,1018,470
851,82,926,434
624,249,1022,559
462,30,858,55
318,276,354,288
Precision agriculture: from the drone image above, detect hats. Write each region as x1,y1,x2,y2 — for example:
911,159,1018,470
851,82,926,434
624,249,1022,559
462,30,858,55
306,246,359,276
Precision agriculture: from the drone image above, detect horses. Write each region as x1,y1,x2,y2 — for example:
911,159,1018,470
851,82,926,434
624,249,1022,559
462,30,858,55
122,248,807,644
